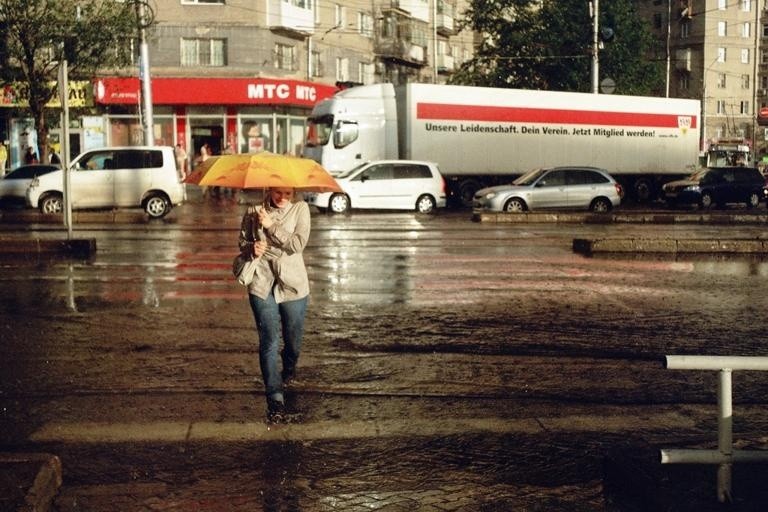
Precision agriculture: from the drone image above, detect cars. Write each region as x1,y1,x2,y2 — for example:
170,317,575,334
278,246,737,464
26,143,188,219
471,165,623,213
0,163,62,209
306,160,447,214
663,166,766,209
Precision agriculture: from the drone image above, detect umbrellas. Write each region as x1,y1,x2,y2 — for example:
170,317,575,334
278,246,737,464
178,151,345,196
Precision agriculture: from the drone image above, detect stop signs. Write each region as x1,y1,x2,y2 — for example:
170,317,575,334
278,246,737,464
759,107,768,118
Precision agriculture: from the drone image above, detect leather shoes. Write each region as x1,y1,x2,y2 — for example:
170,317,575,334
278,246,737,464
280,349,296,386
269,401,284,412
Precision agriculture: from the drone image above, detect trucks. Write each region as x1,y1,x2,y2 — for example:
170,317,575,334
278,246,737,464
301,80,703,208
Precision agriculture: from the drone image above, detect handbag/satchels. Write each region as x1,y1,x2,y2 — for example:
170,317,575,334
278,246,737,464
232,205,266,285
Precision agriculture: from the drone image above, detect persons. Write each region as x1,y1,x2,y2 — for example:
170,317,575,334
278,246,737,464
207,145,212,155
88,158,111,170
223,142,235,155
175,144,187,176
0,142,8,175
237,187,312,424
200,143,208,162
25,146,37,164
50,148,61,163
